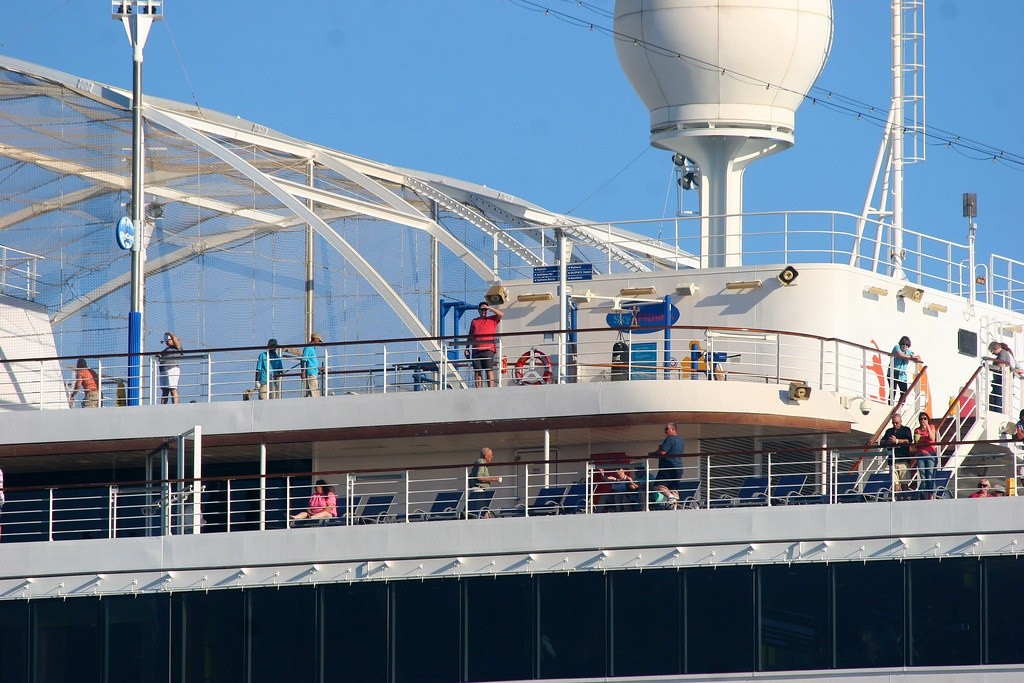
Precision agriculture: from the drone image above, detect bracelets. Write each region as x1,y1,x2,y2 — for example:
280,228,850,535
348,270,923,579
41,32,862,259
896,439,898,444
910,355,912,360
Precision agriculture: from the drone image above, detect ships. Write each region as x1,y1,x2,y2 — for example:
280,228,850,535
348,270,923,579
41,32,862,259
2,0,1024,683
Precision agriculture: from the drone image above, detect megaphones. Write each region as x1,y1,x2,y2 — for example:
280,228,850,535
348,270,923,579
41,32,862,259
484,284,506,305
787,383,811,401
903,285,924,303
778,265,799,285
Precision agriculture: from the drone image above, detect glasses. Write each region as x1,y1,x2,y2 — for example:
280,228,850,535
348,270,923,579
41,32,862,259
978,483,987,487
920,417,927,421
892,421,899,424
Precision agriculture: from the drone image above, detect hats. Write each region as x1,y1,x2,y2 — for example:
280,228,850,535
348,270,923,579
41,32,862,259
268,338,278,348
994,487,1006,493
311,333,323,342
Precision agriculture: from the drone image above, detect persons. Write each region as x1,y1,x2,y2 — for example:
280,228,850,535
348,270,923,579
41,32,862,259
469,448,499,518
156,332,183,405
1012,409,1024,440
284,480,337,519
880,414,912,500
69,359,103,408
300,332,325,397
255,339,283,400
465,302,503,388
968,479,1006,498
596,467,656,513
914,412,936,501
888,336,921,406
988,342,1024,414
648,422,684,510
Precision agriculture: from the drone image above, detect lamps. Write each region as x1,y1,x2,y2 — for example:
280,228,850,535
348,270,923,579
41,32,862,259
571,289,595,303
618,286,658,296
517,292,555,302
927,303,947,312
726,280,763,290
867,287,888,296
677,283,700,295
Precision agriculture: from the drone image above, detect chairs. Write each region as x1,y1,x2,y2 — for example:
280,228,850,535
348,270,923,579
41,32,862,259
444,490,498,519
411,491,465,521
343,495,396,525
811,474,861,503
290,496,364,526
663,480,701,508
862,470,892,501
914,468,953,498
765,470,810,503
545,480,600,515
514,487,567,517
724,473,776,505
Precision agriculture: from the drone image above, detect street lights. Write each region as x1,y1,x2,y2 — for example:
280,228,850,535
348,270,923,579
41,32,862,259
110,0,163,409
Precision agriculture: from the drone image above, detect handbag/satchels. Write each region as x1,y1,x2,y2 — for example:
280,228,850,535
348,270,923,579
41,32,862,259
887,365,900,379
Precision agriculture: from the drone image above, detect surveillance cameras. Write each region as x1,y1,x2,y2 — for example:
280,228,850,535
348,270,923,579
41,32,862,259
859,401,871,415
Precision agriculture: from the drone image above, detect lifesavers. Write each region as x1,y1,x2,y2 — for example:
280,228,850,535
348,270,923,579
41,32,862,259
515,350,552,385
